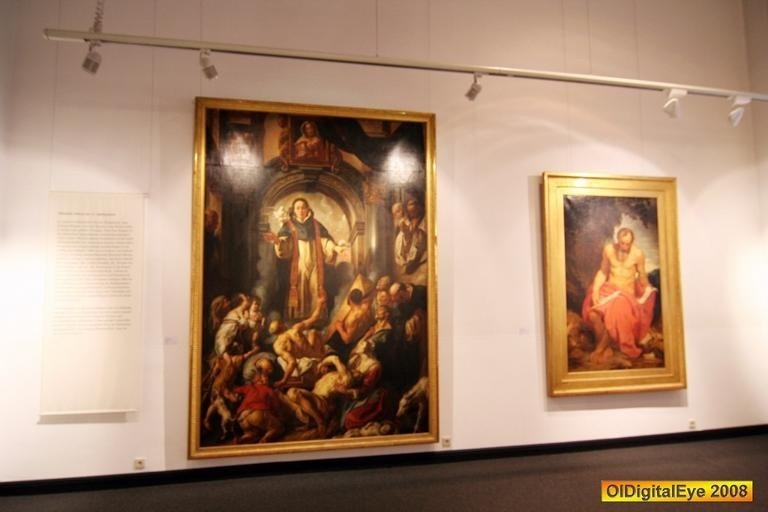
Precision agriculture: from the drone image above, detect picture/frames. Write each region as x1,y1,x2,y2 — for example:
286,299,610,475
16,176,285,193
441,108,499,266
538,170,686,401
185,95,441,464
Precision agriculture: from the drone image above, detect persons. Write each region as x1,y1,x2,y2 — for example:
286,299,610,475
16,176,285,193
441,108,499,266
583,226,657,362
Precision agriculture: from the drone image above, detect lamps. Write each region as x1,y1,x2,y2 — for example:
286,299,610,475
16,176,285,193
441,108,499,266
41,1,768,124
